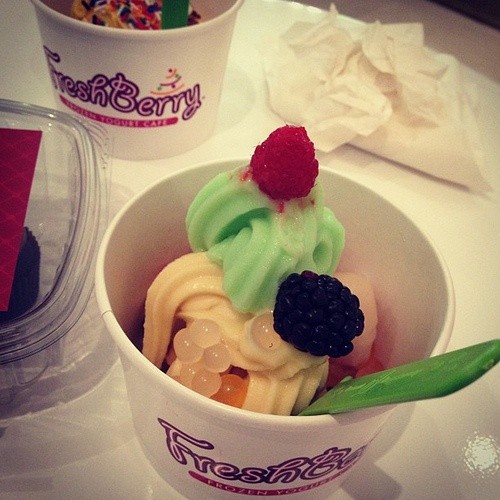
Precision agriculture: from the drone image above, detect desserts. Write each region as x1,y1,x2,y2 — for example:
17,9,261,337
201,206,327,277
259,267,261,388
68,0,201,30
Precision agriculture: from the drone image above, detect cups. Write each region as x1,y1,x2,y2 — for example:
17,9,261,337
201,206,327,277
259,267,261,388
94,157,455,500
32,0,245,163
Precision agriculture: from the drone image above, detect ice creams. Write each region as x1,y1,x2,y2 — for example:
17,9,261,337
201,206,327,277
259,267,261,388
139,123,380,420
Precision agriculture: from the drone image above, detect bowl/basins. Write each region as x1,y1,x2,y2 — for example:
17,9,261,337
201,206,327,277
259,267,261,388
0,96,102,366
